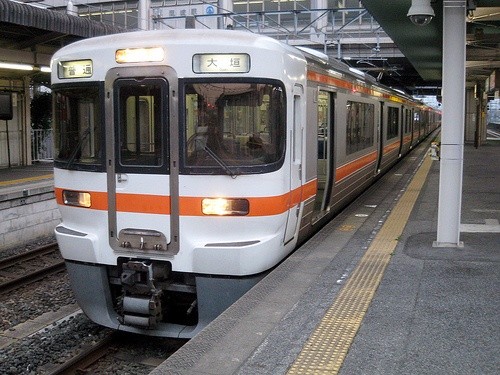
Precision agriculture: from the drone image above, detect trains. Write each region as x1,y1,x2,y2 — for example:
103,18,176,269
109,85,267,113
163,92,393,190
49,27,442,340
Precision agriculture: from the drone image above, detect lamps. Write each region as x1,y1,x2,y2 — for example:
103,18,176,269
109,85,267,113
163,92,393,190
405,0,437,27
